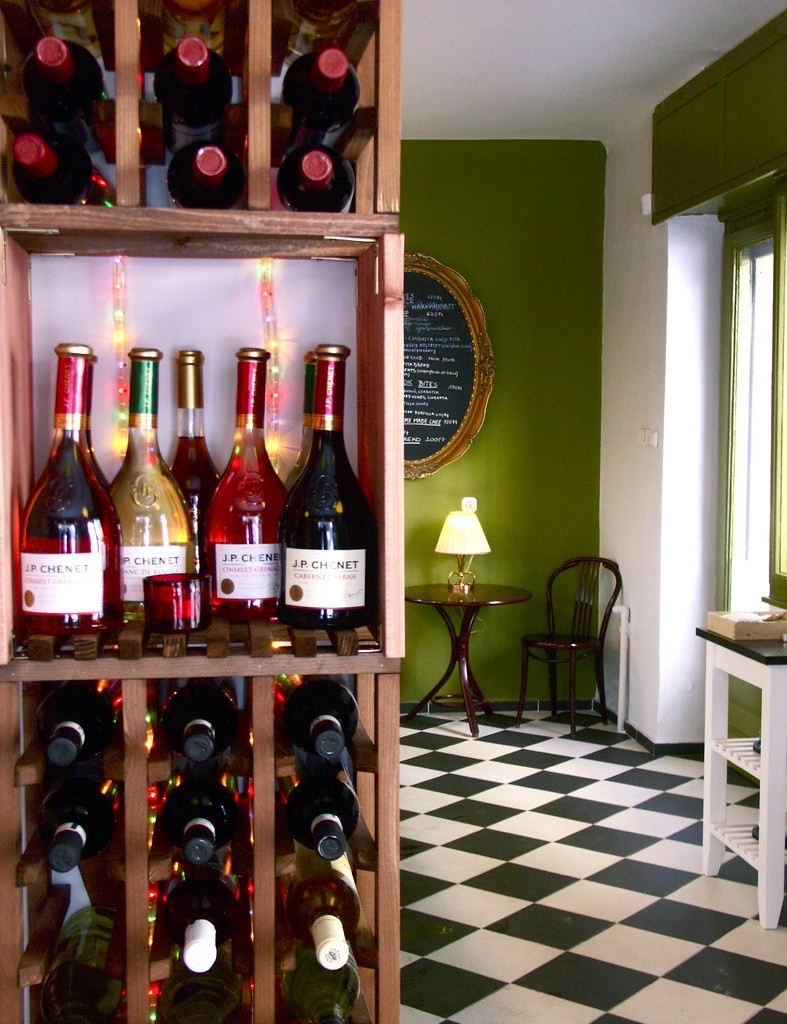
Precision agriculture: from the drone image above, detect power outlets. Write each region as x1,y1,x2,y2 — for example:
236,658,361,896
649,430,658,448
641,193,652,216
462,497,478,512
637,429,649,446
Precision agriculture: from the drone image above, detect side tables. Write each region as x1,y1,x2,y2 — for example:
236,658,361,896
404,583,532,738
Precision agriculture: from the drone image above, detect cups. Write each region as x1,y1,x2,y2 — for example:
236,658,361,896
144,569,213,632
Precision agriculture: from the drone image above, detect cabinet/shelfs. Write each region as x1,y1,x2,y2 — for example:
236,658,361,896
2,0,406,1024
695,626,787,929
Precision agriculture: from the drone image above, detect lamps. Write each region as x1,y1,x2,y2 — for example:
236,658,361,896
434,509,492,591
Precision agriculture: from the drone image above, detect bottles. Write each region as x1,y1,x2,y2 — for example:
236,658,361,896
278,339,372,632
25,679,370,1024
206,346,293,614
157,347,226,537
13,26,364,217
12,339,125,640
102,343,196,621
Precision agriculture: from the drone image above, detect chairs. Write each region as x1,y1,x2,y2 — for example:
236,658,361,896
514,557,623,738
